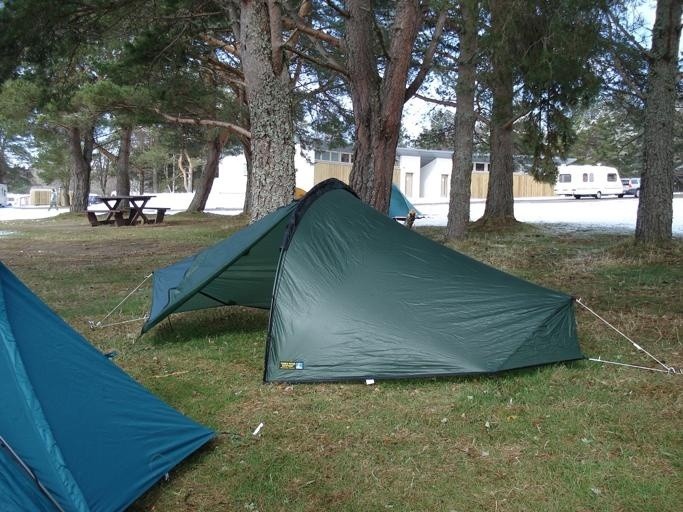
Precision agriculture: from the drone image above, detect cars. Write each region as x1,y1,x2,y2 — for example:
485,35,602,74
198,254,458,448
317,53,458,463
88,196,102,204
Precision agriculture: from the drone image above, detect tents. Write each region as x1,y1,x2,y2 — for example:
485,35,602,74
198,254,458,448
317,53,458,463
0,260,218,511
137,176,584,383
389,181,426,219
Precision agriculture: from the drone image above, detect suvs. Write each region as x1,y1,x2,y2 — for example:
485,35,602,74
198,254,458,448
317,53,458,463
619,177,641,198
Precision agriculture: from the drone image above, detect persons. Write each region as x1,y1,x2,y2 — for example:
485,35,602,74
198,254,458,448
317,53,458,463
46,188,58,211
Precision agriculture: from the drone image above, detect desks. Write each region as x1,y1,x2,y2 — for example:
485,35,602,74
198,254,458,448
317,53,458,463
95,196,156,224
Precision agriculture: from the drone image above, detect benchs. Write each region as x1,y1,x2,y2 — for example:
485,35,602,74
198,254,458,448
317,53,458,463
81,210,121,226
130,207,171,224
